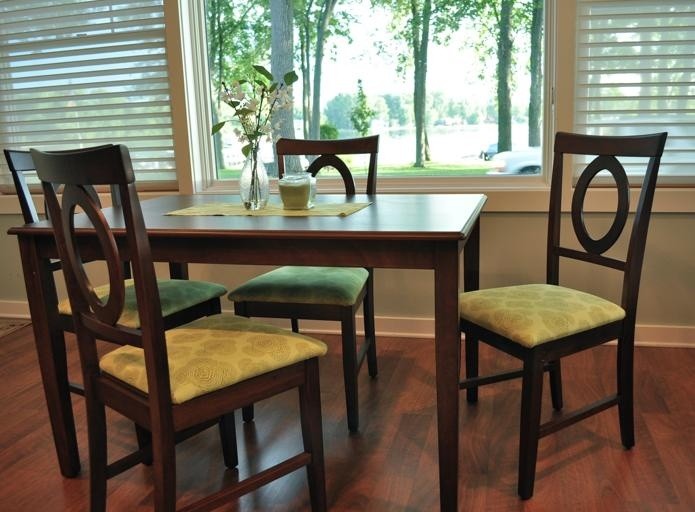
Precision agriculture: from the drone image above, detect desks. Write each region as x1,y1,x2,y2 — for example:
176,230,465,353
8,194,487,512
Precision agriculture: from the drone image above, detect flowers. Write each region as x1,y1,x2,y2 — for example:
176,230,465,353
212,65,297,203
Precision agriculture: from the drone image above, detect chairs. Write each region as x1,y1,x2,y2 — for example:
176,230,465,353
457,131,668,499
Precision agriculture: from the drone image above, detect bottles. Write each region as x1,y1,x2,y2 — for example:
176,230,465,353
279,171,316,210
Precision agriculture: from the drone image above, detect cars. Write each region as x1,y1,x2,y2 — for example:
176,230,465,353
478,142,497,161
484,114,695,177
